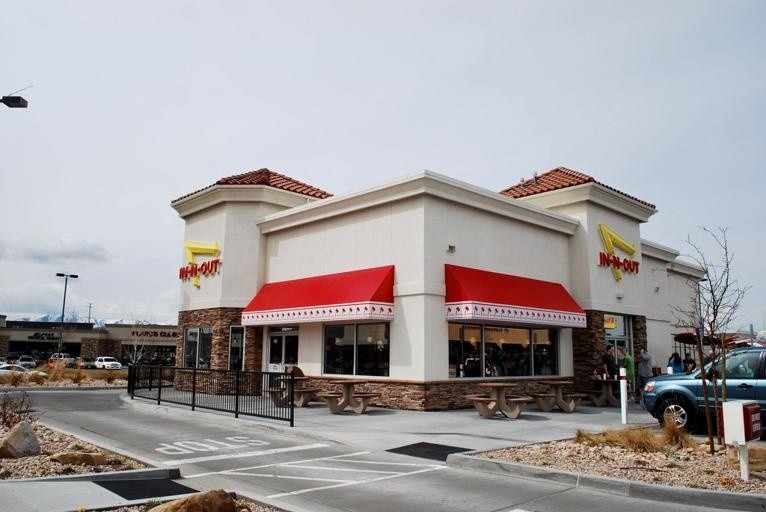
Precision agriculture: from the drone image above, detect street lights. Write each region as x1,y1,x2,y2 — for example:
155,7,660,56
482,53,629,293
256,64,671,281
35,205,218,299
56,272,79,352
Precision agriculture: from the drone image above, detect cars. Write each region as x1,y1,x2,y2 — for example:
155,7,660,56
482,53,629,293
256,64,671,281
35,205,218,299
94,356,122,370
15,355,37,368
0,363,49,381
641,345,766,441
50,352,95,369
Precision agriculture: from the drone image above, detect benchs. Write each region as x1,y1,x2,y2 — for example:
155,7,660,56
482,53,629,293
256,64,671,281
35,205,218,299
316,392,381,414
263,386,323,407
462,391,620,419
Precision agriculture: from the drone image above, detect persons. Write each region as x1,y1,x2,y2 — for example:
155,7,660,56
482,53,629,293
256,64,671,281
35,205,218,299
703,350,714,365
638,349,653,387
603,345,618,379
682,353,696,374
619,346,635,402
667,352,684,374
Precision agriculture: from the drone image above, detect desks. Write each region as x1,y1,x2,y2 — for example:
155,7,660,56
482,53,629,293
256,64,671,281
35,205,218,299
329,379,369,399
479,379,620,402
278,376,309,392
